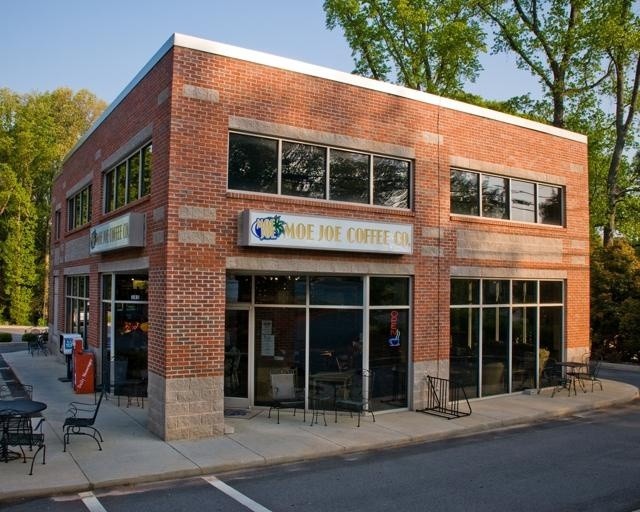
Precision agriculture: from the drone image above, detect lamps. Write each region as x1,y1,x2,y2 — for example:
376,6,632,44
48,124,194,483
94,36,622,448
274,279,294,308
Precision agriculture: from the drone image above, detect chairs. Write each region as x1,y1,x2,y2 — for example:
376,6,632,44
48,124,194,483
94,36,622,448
266,366,305,424
334,371,376,427
62,389,105,451
0,381,45,476
537,353,604,398
225,346,241,390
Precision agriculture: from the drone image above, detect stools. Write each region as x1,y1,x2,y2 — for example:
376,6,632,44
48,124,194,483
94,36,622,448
115,379,146,407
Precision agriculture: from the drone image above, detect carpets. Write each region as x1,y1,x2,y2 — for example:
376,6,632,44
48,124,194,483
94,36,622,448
224,407,263,420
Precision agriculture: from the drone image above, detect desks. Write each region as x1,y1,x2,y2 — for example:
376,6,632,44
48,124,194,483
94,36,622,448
0,398,48,451
307,395,330,427
